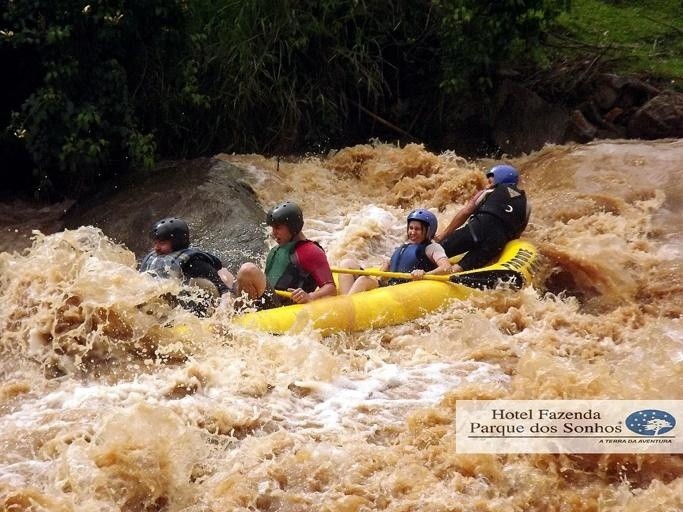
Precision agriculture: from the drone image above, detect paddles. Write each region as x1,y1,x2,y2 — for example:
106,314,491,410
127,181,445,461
329,267,523,293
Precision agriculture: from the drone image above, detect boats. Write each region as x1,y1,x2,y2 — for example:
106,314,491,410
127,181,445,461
149,238,552,362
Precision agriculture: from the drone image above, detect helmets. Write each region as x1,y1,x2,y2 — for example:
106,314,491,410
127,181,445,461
266,202,303,234
152,216,189,251
486,165,518,189
407,208,437,241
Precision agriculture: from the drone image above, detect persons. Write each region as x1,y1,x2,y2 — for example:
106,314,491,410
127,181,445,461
435,164,531,274
138,216,235,319
338,209,451,294
238,202,336,311
